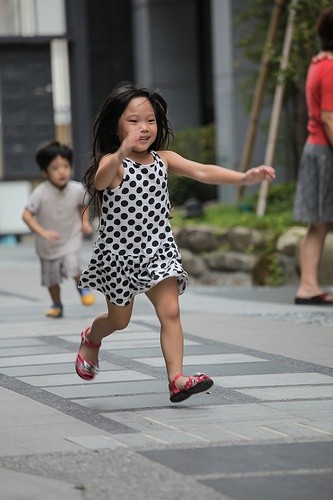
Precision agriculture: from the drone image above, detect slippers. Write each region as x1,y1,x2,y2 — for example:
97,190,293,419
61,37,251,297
295,293,333,305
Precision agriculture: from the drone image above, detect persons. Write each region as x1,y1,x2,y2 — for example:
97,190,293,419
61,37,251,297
292,7,333,306
75,85,276,402
22,142,94,318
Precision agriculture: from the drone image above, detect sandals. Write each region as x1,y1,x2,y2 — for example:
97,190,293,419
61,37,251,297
75,327,102,381
169,372,214,402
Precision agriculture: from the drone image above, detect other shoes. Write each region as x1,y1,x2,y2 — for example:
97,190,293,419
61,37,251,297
77,287,95,306
45,304,63,318
